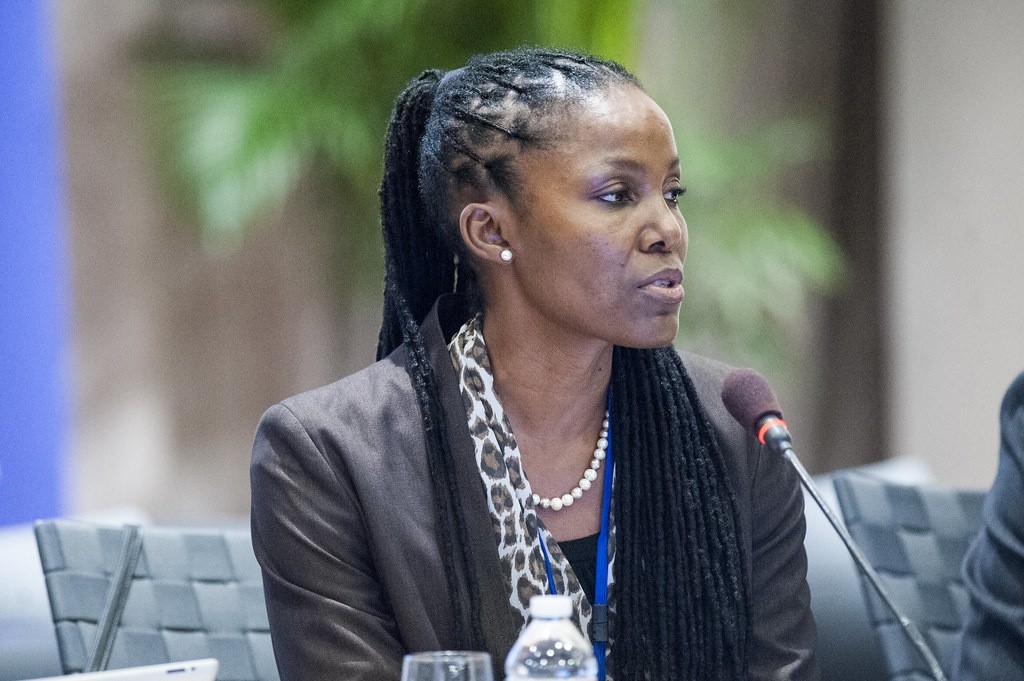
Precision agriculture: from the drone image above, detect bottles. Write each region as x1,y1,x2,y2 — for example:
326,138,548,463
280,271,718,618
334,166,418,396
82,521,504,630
504,594,598,680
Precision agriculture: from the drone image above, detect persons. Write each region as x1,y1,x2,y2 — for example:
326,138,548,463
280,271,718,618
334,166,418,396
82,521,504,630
249,48,822,681
949,370,1024,681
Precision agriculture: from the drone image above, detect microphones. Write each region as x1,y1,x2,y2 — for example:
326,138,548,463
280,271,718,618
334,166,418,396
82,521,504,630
719,367,948,681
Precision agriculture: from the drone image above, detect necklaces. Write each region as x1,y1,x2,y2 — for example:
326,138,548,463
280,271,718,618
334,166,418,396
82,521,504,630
533,410,610,509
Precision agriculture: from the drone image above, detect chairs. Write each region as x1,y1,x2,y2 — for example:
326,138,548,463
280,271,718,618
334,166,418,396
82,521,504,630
32,516,279,681
834,472,996,681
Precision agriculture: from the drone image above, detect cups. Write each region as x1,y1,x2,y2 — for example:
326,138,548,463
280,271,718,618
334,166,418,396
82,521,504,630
400,650,494,680
